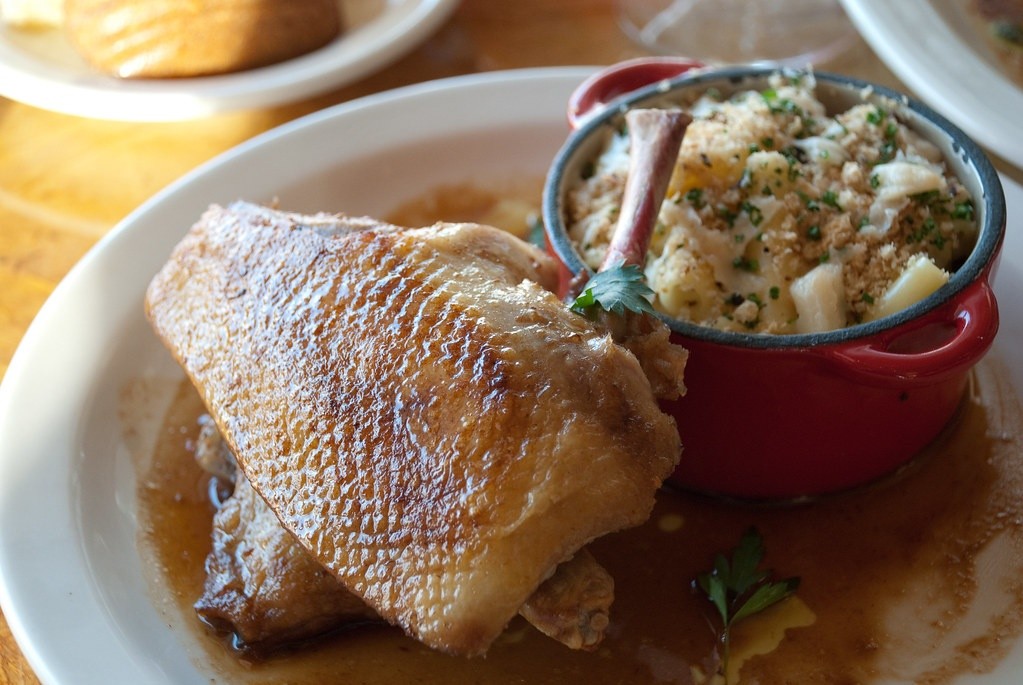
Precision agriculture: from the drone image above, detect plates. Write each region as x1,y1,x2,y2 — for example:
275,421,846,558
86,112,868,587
841,0,1022,171
1,64,1022,685
1,0,458,125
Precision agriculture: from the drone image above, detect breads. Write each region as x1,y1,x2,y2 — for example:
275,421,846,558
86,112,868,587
61,0,341,80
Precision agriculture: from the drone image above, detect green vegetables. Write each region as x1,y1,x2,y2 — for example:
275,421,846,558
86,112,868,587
572,64,980,683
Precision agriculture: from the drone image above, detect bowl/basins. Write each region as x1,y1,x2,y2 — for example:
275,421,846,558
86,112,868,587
540,53,1007,497
